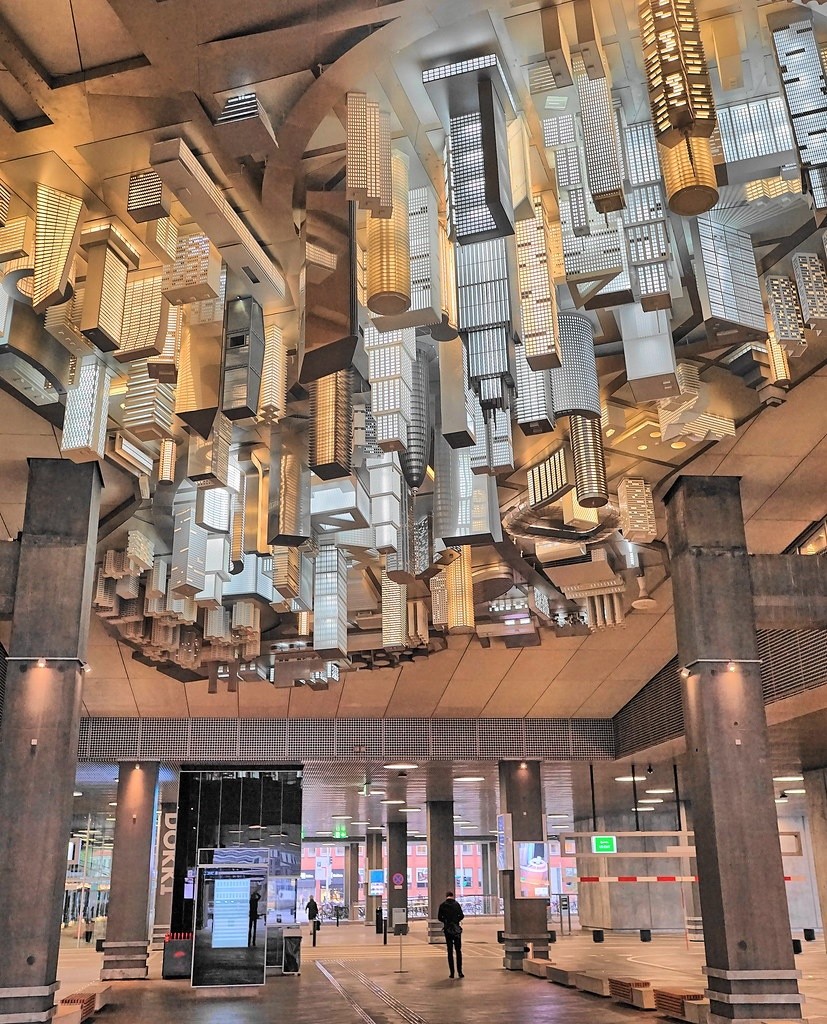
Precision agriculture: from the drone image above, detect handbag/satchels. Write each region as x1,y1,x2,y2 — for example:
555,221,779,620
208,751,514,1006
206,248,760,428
447,922,462,938
316,921,321,931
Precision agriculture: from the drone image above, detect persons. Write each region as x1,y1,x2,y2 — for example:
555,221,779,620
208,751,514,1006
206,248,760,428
437,891,465,979
305,895,318,935
248,892,262,947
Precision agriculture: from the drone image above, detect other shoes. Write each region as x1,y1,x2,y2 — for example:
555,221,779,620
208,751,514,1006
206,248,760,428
248,942,251,945
310,933,313,935
252,941,256,946
449,974,454,978
459,974,464,978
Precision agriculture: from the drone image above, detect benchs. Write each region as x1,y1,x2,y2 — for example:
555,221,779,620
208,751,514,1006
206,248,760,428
546,965,586,987
608,975,656,1009
80,985,112,1011
576,972,610,996
653,988,711,1024
51,992,96,1024
523,958,556,978
